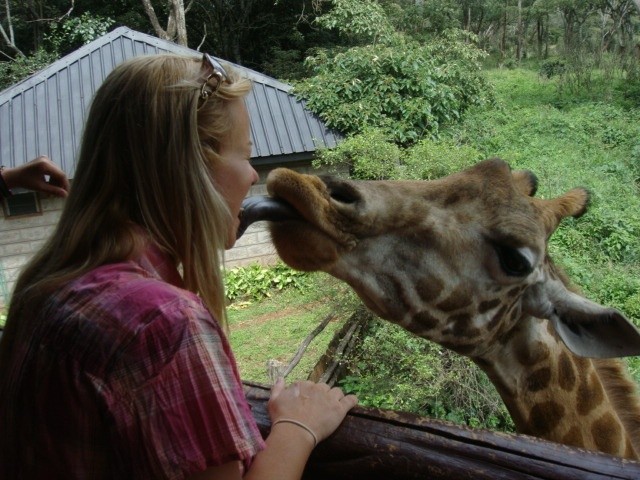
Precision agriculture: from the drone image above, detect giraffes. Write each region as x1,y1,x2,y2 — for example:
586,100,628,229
237,159,640,479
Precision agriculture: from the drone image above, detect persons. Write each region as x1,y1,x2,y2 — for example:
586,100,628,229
0,51,360,477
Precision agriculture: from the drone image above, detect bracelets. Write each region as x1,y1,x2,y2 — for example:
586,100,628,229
272,418,317,446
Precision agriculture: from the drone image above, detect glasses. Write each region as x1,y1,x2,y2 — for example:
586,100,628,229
198,52,231,114
